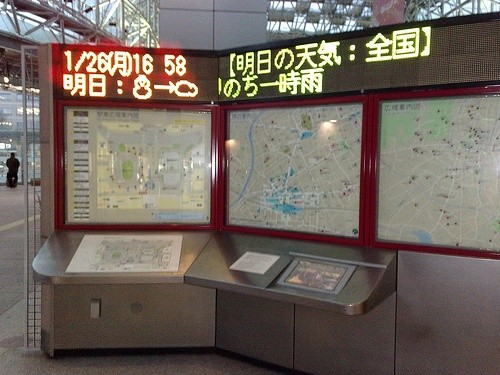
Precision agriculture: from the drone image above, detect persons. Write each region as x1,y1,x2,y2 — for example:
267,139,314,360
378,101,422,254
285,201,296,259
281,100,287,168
5,153,20,187
